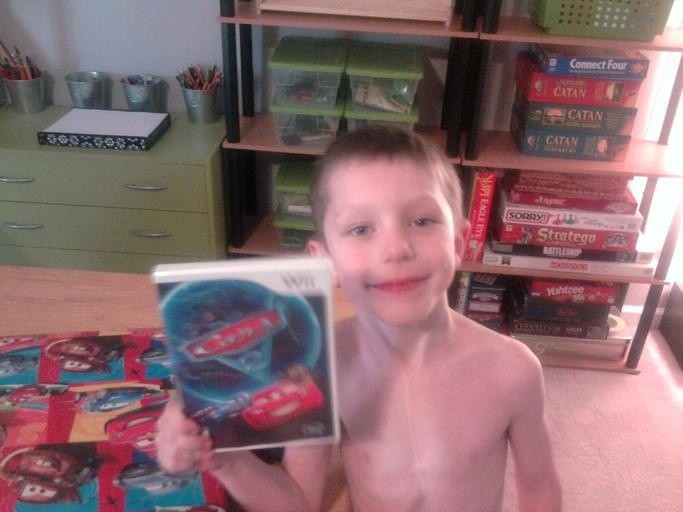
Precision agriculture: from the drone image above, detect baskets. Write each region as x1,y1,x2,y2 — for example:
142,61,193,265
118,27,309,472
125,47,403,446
533,0,671,39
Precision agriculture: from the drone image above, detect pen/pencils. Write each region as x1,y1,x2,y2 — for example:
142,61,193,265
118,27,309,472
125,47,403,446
175,62,223,90
122,74,152,85
0,40,41,80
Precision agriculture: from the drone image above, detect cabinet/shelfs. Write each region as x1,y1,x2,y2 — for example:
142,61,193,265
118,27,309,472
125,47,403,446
1,105,233,274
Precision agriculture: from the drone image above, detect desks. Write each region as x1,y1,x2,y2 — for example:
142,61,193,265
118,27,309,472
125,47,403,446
1,264,232,512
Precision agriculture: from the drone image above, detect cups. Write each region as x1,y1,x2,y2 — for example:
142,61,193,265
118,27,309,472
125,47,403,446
181,87,220,126
121,73,170,113
65,71,111,113
5,75,44,115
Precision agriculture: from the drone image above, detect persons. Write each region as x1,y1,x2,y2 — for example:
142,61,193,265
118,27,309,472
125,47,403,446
152,127,565,512
